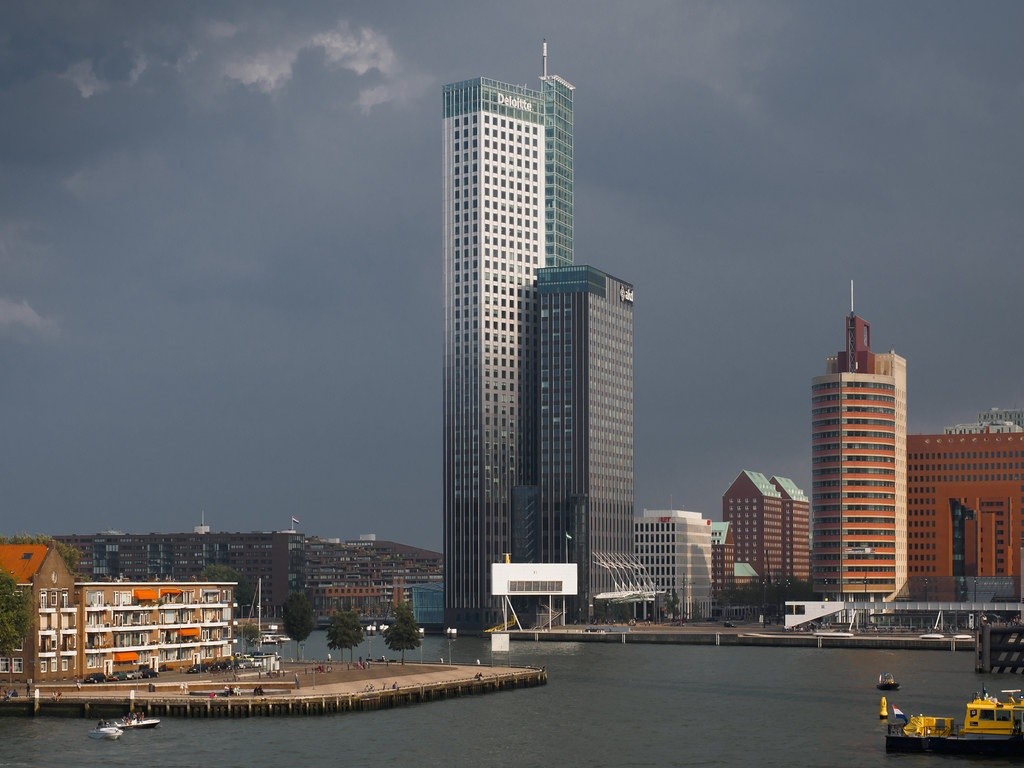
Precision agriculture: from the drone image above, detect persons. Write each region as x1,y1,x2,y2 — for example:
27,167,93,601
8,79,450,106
225,685,241,696
76,679,81,688
474,672,482,679
254,685,264,696
98,719,106,727
393,681,398,689
26,683,30,697
3,689,18,701
53,689,62,702
358,659,370,669
628,618,636,625
127,711,145,721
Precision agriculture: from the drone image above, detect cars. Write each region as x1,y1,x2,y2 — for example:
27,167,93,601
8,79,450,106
84,668,159,684
209,659,253,672
233,651,282,667
187,664,208,673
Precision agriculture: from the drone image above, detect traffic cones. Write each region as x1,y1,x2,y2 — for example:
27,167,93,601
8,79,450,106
879,696,888,720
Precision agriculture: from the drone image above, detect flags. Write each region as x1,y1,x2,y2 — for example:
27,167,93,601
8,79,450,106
292,516,299,523
893,706,908,722
566,534,572,540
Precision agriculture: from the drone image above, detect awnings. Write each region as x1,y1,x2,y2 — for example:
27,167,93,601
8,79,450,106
134,590,157,599
115,652,139,661
177,629,199,636
161,589,181,599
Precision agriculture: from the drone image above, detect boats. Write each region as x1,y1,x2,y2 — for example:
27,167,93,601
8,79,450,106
101,717,161,730
876,671,900,690
245,624,292,645
88,728,124,741
